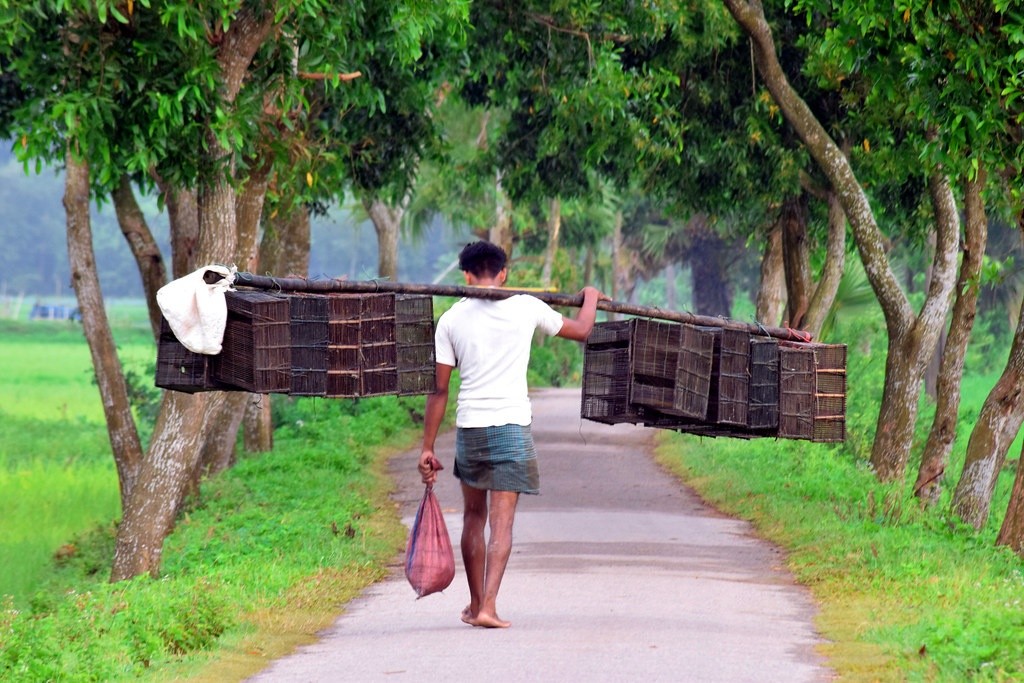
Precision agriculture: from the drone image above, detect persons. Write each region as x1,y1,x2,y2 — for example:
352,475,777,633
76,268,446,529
418,240,614,629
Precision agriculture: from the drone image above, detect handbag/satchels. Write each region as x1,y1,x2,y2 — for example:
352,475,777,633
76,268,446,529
156,265,237,356
404,458,456,598
